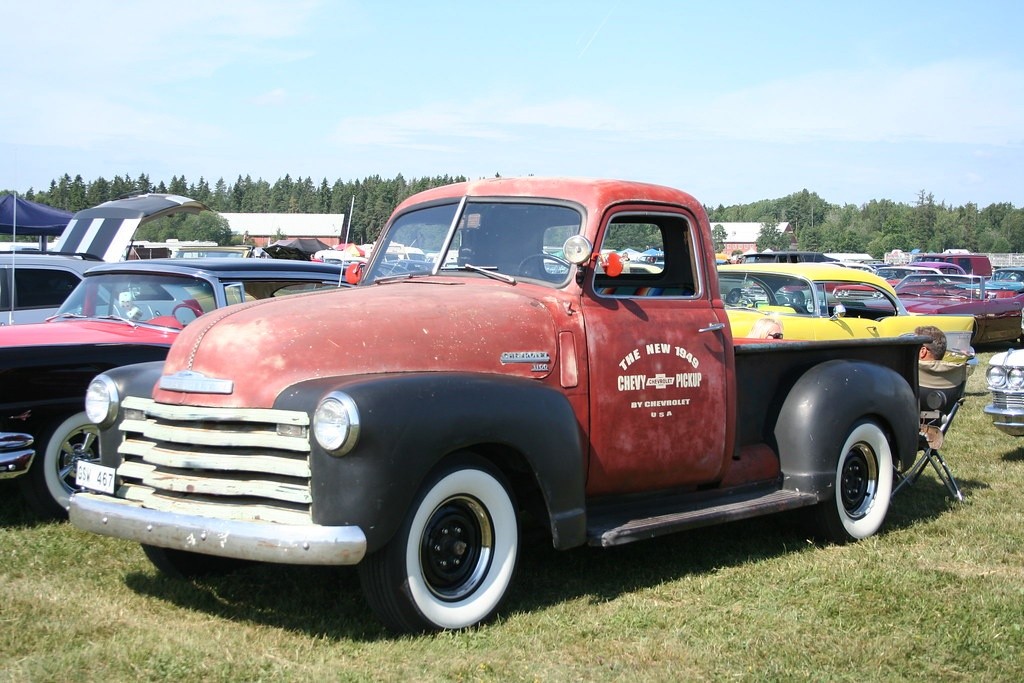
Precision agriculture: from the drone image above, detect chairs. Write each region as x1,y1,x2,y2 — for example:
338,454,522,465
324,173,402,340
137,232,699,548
890,383,967,505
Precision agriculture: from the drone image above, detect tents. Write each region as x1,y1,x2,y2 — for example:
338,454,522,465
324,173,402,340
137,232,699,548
262,239,365,261
741,248,773,255
553,248,665,261
0,194,76,252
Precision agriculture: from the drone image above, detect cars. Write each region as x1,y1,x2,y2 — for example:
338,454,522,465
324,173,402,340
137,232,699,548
0,192,1023,332
1,261,395,519
709,262,976,374
982,349,1022,436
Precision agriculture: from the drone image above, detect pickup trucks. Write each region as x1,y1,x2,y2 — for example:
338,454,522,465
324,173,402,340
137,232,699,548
65,179,941,638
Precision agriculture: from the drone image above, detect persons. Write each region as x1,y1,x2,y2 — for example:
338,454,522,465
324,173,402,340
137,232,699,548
791,290,805,307
748,319,783,339
727,288,742,304
914,326,965,388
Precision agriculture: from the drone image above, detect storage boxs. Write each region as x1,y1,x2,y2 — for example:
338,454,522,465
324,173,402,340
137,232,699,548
944,330,973,350
